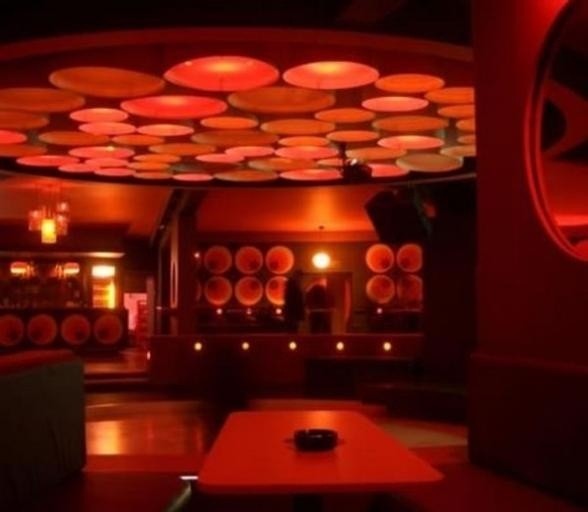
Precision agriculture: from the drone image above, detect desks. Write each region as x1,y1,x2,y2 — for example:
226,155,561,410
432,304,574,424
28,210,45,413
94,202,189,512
196,409,446,511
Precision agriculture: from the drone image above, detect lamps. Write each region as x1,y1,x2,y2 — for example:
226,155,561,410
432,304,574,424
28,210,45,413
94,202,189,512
41,189,57,245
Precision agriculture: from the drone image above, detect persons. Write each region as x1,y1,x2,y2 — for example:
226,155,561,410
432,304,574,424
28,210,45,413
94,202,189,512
283,269,307,335
